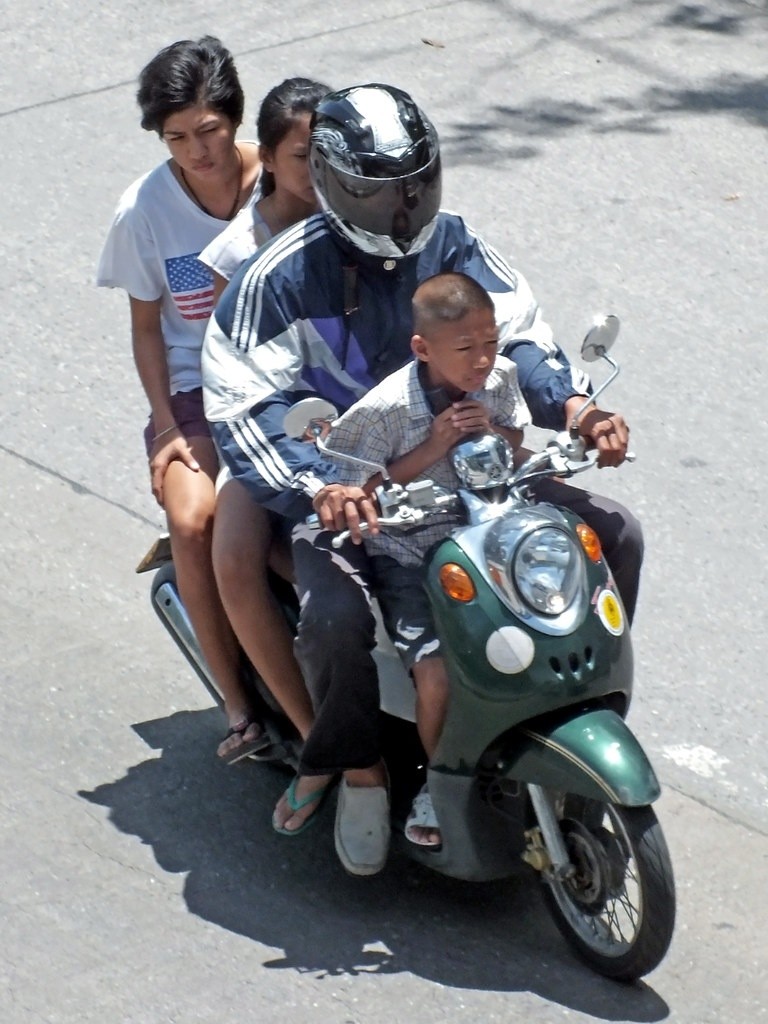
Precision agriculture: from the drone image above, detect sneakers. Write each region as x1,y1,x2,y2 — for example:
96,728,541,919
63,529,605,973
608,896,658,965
333,753,391,881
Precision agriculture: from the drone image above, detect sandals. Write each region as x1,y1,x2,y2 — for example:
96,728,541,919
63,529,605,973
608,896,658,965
220,714,273,767
271,772,341,837
404,779,445,853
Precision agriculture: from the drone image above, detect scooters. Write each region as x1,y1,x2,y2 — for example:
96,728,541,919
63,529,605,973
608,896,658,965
136,314,677,983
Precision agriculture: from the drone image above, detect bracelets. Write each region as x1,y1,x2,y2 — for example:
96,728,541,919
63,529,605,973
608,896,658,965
150,422,178,443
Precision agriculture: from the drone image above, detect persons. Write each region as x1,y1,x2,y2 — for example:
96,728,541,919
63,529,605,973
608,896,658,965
102,35,645,882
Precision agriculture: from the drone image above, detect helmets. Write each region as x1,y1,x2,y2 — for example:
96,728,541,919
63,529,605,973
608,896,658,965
310,78,446,258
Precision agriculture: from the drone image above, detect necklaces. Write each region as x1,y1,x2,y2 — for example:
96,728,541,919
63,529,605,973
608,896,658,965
179,145,243,221
